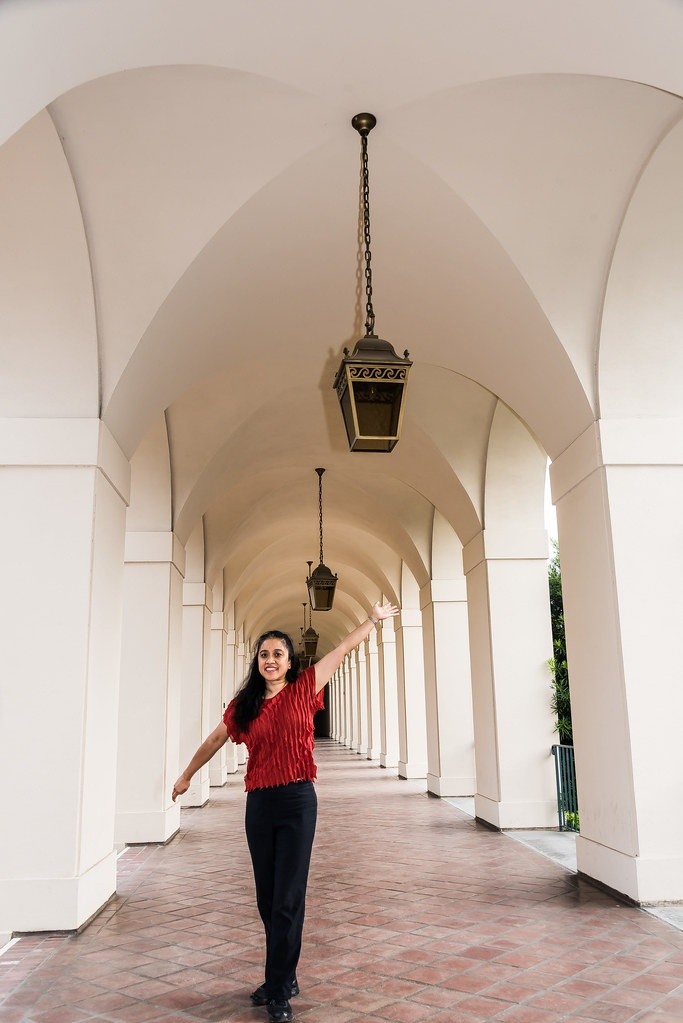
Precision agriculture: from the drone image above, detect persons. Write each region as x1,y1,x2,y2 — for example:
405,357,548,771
171,600,402,1023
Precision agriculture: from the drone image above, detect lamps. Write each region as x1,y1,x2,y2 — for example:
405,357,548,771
305,467,339,612
302,561,319,658
332,113,415,453
297,602,311,671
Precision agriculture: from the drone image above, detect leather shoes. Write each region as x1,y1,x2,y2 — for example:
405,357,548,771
250,978,299,1005
264,994,295,1023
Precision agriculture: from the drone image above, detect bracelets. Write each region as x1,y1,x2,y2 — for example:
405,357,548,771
368,616,378,625
